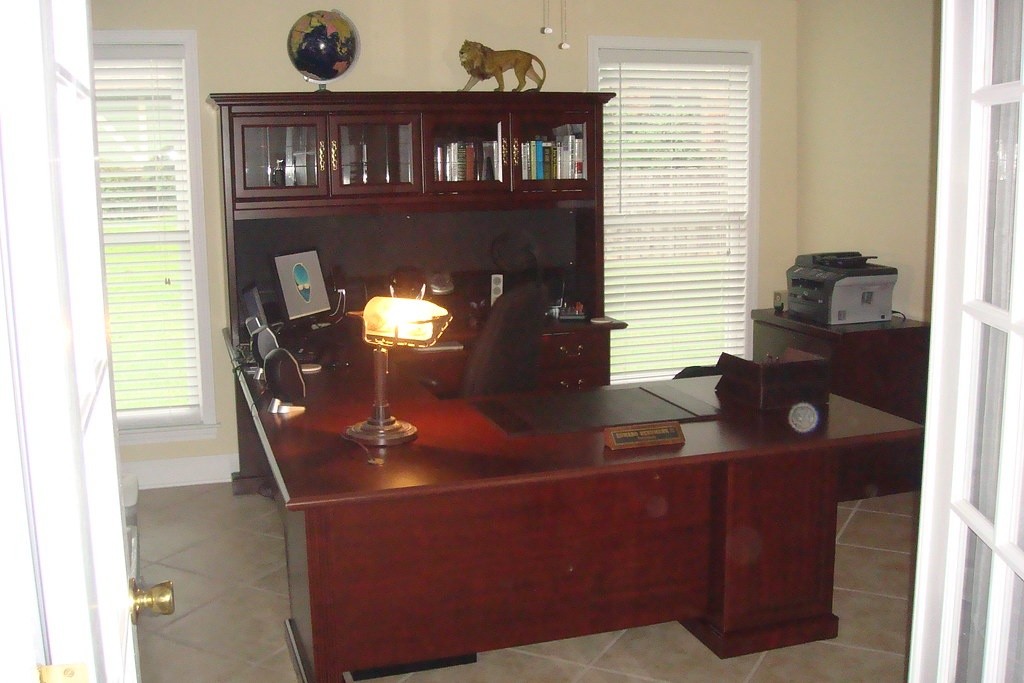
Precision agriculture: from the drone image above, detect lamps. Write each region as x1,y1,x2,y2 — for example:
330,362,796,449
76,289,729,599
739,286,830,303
345,296,454,446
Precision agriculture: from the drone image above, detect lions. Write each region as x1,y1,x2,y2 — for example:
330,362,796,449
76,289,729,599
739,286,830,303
457,38,547,93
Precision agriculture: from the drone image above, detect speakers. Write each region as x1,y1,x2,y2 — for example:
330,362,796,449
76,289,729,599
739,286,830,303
250,326,307,413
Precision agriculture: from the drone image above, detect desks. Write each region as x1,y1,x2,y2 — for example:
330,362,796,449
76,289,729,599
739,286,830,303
222,326,925,683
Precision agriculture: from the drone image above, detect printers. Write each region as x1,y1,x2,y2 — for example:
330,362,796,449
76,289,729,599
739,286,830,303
787,251,899,326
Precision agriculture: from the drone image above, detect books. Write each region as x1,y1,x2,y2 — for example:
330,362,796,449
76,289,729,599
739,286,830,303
521,134,585,180
433,140,499,182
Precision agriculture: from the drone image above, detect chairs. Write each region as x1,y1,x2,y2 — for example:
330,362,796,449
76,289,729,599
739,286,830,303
419,268,544,398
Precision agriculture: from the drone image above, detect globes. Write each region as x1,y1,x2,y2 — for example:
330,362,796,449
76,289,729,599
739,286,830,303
287,7,363,91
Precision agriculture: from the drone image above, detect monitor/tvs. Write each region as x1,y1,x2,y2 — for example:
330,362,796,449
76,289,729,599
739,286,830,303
239,280,267,326
270,248,332,335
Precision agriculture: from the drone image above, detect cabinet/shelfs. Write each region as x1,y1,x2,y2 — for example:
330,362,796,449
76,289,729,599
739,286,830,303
751,308,932,501
210,92,628,390
